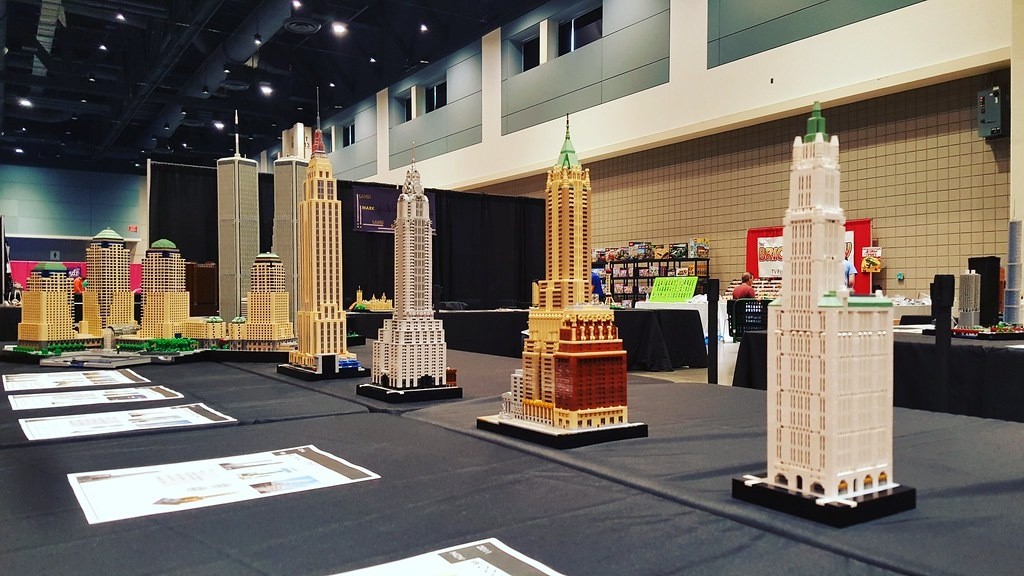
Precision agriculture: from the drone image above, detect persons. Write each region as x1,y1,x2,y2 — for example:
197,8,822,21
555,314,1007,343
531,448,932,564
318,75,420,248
844,256,858,288
591,272,604,303
733,272,756,299
